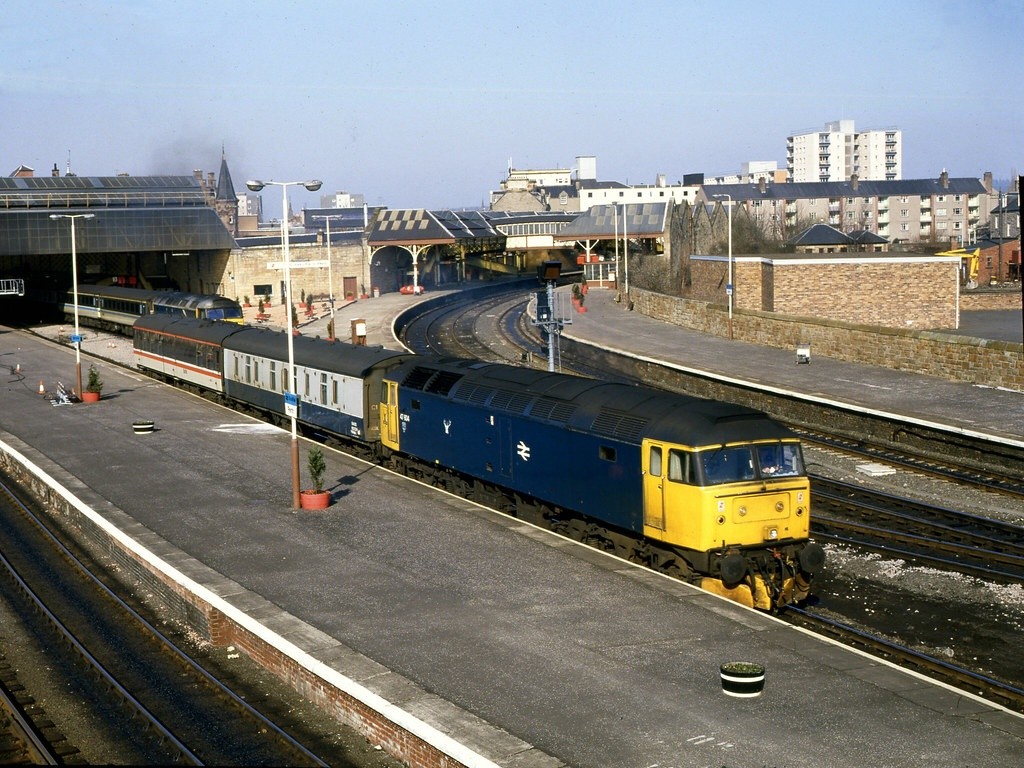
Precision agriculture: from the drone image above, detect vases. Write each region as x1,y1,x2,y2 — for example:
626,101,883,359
719,661,765,697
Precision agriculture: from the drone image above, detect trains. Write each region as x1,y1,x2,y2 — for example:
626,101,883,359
28,282,244,338
132,311,825,615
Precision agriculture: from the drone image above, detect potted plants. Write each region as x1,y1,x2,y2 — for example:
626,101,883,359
346,290,355,301
82,364,103,402
299,289,307,308
242,295,250,307
305,296,313,316
264,291,271,308
571,275,589,313
256,298,272,318
360,284,368,299
310,295,314,309
300,447,329,509
324,322,338,341
291,306,300,335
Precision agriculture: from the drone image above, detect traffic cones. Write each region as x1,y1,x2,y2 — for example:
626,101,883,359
15,364,22,373
38,380,46,394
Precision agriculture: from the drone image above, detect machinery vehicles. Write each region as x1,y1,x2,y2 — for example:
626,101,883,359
934,248,980,290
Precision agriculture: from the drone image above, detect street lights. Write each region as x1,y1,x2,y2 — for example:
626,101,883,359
712,194,734,340
605,204,619,293
244,179,323,510
50,214,96,401
312,214,345,317
612,201,629,307
